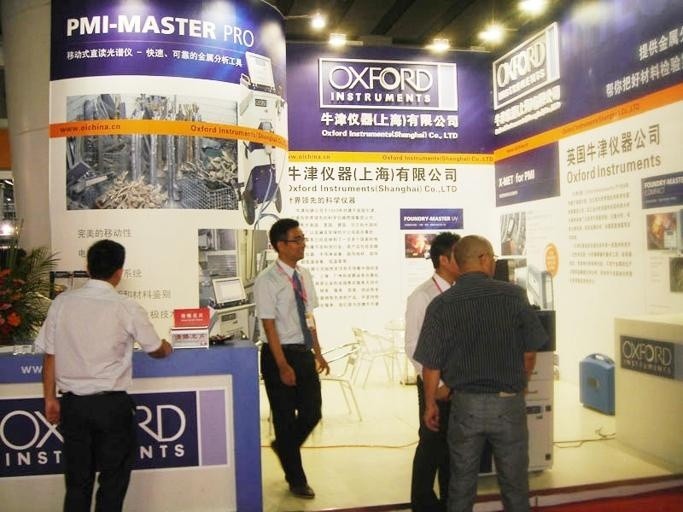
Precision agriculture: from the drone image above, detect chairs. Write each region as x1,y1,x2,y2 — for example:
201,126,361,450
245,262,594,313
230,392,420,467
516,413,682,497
261,319,421,447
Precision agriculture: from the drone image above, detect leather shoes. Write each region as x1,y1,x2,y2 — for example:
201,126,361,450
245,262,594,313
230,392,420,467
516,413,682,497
291,484,314,498
271,441,279,458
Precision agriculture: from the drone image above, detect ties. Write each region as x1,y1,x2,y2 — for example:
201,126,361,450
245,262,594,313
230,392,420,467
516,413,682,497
291,270,313,353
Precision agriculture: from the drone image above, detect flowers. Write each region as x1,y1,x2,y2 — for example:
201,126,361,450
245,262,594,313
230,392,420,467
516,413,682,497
0,245,66,344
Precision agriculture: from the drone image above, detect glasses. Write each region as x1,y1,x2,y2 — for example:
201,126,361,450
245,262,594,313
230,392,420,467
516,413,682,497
284,236,308,245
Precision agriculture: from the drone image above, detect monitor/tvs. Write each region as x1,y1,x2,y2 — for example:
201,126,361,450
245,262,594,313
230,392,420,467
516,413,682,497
211,276,246,304
246,52,275,89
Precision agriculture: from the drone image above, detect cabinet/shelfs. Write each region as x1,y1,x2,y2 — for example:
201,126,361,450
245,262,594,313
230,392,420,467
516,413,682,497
476,301,556,476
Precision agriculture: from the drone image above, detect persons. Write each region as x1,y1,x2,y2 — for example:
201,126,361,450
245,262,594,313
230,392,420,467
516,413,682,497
409,233,551,511
28,236,177,511
250,216,332,502
401,230,463,511
